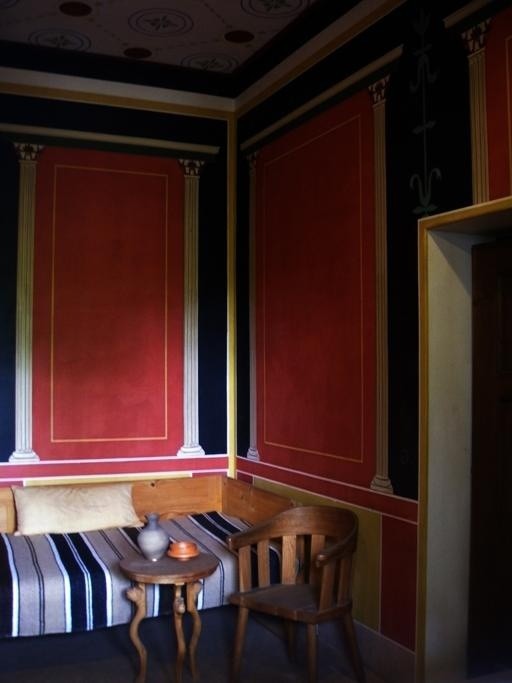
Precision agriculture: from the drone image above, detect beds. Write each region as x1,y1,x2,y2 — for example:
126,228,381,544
0,472,326,644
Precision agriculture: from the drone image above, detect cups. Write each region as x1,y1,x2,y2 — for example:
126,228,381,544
167,537,199,563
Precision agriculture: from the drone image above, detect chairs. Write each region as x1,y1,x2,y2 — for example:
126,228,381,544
225,504,369,682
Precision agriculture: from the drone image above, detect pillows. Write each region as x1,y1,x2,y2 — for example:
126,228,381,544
11,483,146,539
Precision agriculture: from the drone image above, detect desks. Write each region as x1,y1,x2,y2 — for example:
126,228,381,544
119,551,220,683
